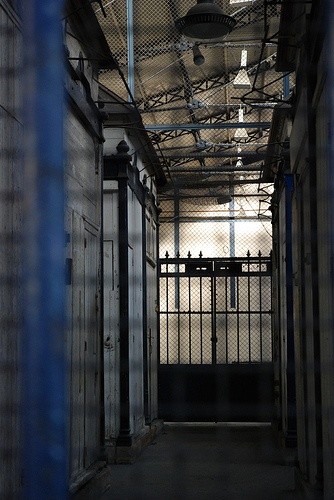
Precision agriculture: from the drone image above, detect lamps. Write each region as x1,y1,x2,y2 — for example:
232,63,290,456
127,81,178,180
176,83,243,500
236,144,243,167
193,45,205,66
174,0,237,43
233,45,252,89
234,104,249,140
